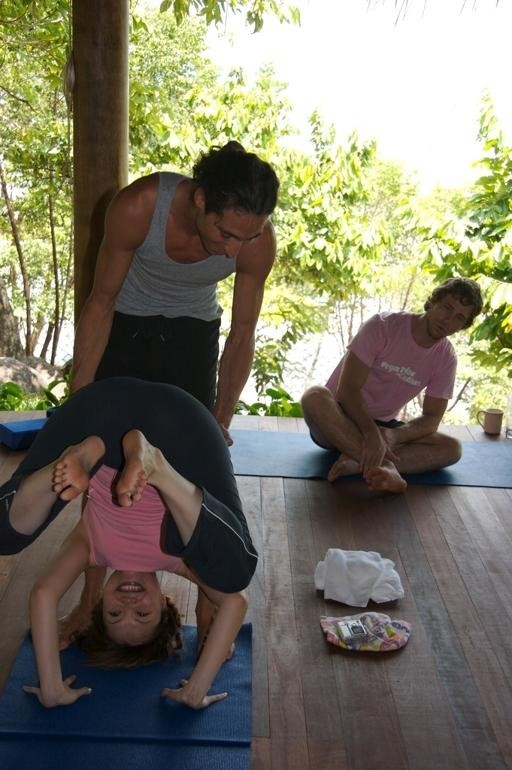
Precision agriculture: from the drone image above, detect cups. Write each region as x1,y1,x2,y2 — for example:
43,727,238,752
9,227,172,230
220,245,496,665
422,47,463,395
476,407,503,436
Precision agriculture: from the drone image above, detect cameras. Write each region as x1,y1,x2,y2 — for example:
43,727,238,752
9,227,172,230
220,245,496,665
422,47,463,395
336,620,368,645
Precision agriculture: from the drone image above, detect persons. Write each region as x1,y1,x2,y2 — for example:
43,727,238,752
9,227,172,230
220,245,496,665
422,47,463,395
300,275,483,496
0,374,259,708
55,139,280,659
